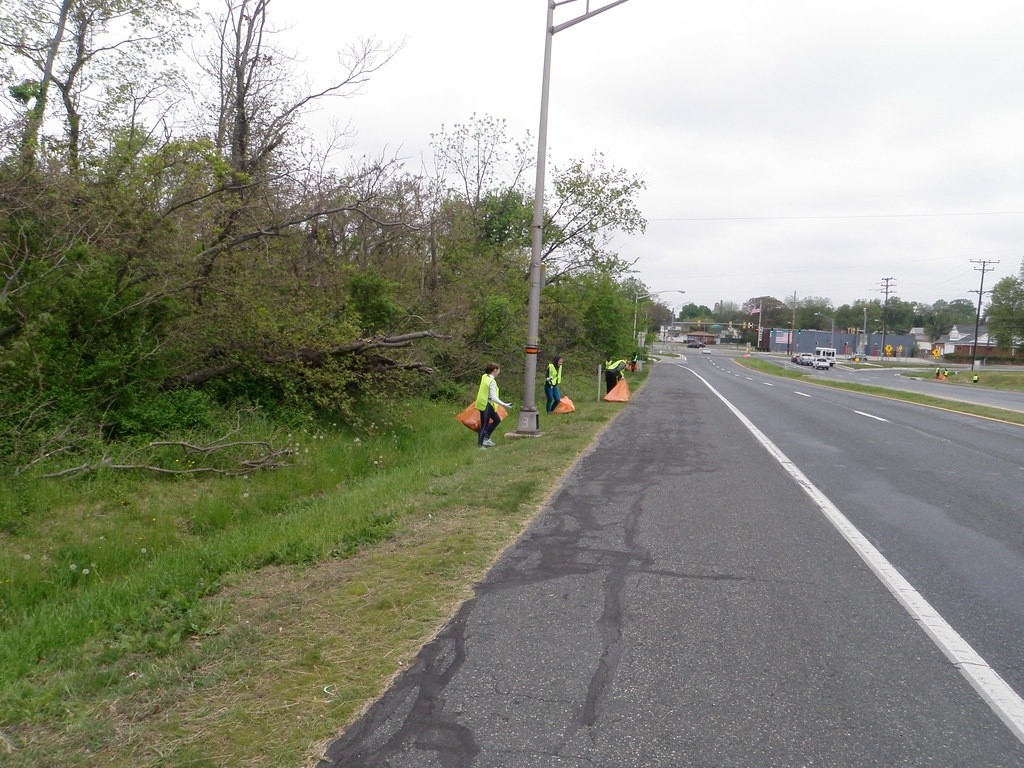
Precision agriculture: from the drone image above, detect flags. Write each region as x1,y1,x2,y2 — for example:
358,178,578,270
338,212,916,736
751,302,760,315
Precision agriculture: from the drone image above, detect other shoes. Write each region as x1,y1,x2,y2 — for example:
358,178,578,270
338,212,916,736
477,445,487,451
483,438,496,447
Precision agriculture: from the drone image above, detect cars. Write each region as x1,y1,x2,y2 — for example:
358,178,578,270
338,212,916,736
696,341,705,347
687,342,700,348
791,354,798,362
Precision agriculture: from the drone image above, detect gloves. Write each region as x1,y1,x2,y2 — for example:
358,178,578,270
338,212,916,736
504,403,512,409
547,377,553,382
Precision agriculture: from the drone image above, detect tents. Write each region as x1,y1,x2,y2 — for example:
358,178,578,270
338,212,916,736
687,331,714,343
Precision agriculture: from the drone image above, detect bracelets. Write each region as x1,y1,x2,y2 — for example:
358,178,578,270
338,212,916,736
503,403,506,406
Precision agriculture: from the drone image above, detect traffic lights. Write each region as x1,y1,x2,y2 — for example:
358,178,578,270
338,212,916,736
770,328,773,332
798,328,801,335
756,327,758,332
750,323,753,329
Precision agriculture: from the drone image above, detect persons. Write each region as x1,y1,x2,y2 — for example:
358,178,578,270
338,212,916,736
943,368,949,377
973,374,978,383
936,367,940,375
605,352,638,394
545,357,563,413
474,363,512,450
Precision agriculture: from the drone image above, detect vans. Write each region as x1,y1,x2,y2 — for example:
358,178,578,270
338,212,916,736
815,347,836,367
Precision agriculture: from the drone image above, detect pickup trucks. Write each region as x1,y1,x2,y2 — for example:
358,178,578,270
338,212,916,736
797,353,812,365
813,358,830,370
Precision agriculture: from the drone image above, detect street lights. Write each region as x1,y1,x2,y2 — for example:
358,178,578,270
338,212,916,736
633,290,686,341
814,313,834,348
670,301,693,348
